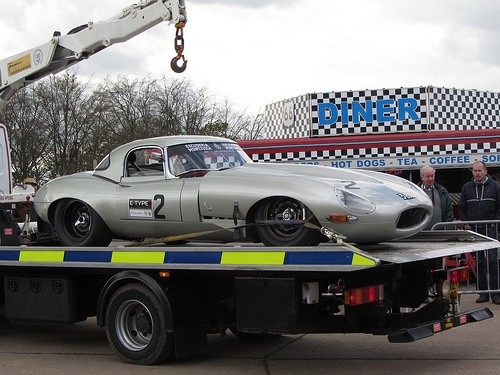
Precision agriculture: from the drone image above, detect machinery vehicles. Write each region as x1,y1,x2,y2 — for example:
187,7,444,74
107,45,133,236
0,0,500,365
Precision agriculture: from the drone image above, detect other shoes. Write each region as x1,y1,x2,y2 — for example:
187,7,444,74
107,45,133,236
492,298,500,305
476,296,490,303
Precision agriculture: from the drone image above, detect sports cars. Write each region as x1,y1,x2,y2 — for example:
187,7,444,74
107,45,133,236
33,133,434,247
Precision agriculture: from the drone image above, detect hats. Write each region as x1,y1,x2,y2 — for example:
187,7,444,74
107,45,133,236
23,178,38,185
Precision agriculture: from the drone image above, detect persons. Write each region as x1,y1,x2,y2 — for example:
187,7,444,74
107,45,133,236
457,161,500,304
416,166,454,295
